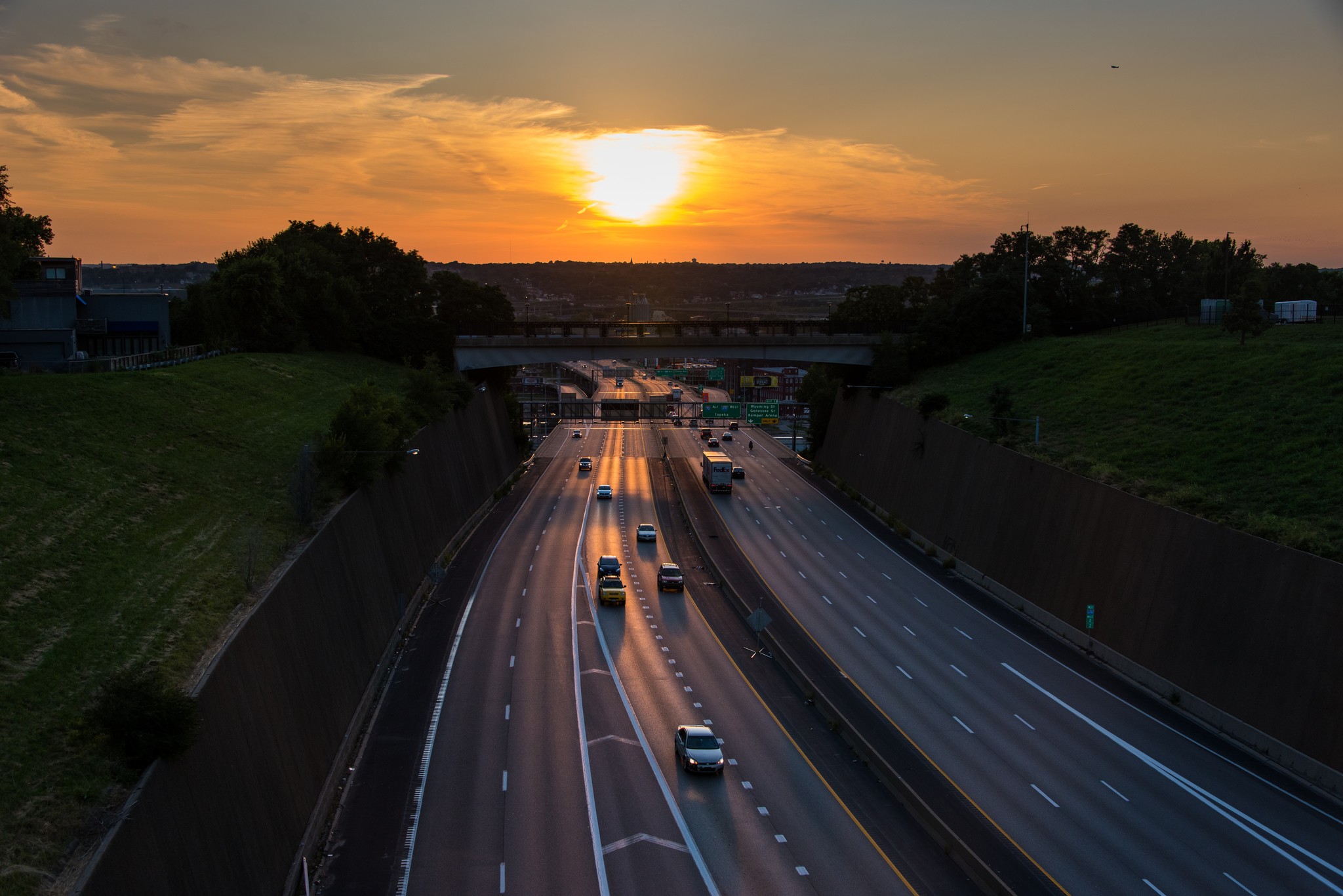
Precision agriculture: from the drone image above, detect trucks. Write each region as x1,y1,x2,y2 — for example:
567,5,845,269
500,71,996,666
670,387,681,402
700,451,733,495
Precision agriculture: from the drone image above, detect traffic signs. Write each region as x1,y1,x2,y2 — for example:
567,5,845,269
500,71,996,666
708,370,725,380
746,403,779,424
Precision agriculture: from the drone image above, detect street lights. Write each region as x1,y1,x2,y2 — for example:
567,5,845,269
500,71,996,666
626,303,632,337
827,303,832,336
545,369,573,439
964,414,1040,445
764,372,796,452
737,366,745,417
725,303,731,337
525,304,530,338
1017,224,1029,334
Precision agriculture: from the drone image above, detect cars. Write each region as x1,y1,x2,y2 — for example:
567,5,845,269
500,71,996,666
680,389,684,394
597,556,622,579
596,485,613,499
732,467,745,478
636,523,658,542
565,360,673,386
666,404,738,447
674,724,725,775
572,430,582,438
673,384,679,387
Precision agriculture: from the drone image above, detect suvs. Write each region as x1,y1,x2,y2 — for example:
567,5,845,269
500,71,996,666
657,563,685,592
598,575,627,606
578,456,593,470
